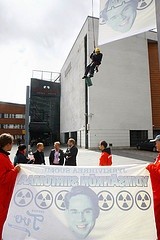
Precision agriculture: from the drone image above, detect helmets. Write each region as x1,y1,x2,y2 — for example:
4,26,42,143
95,47,100,53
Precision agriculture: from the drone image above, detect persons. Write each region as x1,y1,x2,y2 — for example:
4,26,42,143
63,186,100,240
98,141,112,166
81,48,103,79
0,132,21,240
13,137,78,168
145,135,160,240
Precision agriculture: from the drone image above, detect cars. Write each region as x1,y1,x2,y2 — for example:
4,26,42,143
137,139,156,152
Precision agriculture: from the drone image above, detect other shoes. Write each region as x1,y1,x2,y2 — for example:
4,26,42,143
89,75,93,78
82,76,87,79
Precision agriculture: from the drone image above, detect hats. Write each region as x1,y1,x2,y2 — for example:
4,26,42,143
149,134,160,142
18,143,27,151
